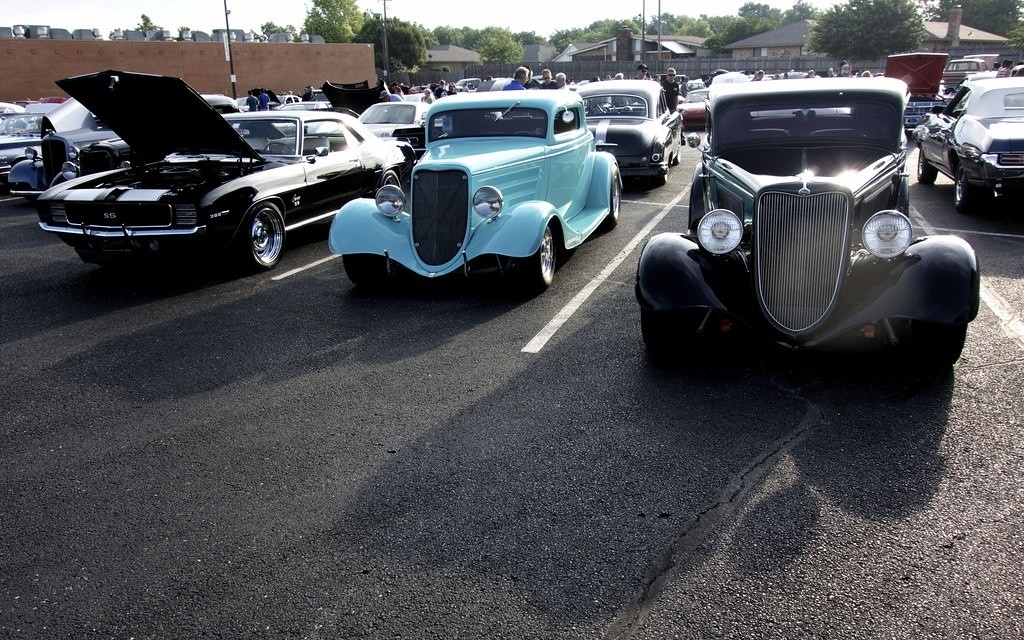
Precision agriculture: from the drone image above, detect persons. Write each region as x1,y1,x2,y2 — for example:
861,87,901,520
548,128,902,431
589,61,888,96
503,65,530,92
302,86,317,102
539,69,559,89
246,90,259,111
996,59,1023,77
378,76,494,106
659,67,679,116
554,72,569,89
521,65,542,89
258,88,272,112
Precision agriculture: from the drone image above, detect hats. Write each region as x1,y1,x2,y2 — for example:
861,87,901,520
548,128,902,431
636,64,648,70
378,91,390,99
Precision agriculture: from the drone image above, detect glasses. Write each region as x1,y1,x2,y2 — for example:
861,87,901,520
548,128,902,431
638,70,648,72
667,74,675,77
543,76,552,79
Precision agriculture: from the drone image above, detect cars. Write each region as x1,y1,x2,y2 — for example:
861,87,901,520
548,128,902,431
0,68,519,271
942,58,988,82
884,52,950,134
679,87,710,129
949,72,998,97
633,76,982,365
574,80,683,185
911,76,1024,214
328,89,625,296
